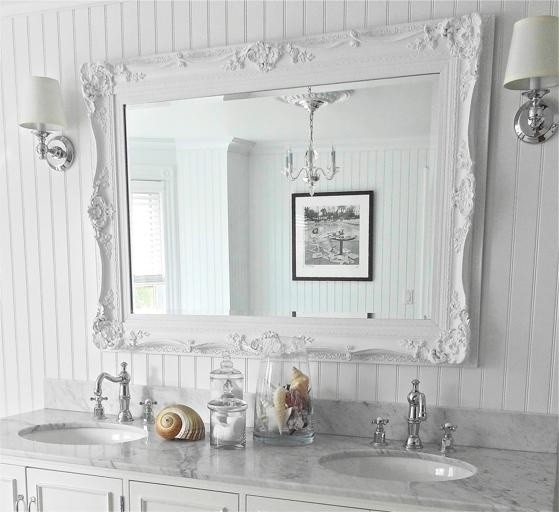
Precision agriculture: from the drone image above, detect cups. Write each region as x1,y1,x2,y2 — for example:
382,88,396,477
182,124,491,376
252,335,314,447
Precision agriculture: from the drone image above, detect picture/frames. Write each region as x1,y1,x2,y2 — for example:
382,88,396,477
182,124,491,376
292,191,374,280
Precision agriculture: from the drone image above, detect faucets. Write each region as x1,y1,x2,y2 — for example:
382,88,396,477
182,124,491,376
404,379,426,449
94,362,134,422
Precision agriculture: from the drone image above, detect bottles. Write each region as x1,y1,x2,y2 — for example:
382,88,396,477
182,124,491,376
205,361,248,450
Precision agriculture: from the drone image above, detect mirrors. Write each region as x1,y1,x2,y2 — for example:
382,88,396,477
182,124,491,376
79,12,496,365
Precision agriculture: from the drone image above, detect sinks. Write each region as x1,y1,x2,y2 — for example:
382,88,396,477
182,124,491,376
21,426,144,444
320,454,475,480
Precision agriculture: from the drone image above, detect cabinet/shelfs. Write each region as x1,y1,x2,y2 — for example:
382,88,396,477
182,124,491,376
130,480,239,511
0,464,123,512
246,495,370,511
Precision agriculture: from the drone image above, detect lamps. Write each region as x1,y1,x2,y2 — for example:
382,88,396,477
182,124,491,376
503,14,558,144
277,89,354,196
19,75,74,172
